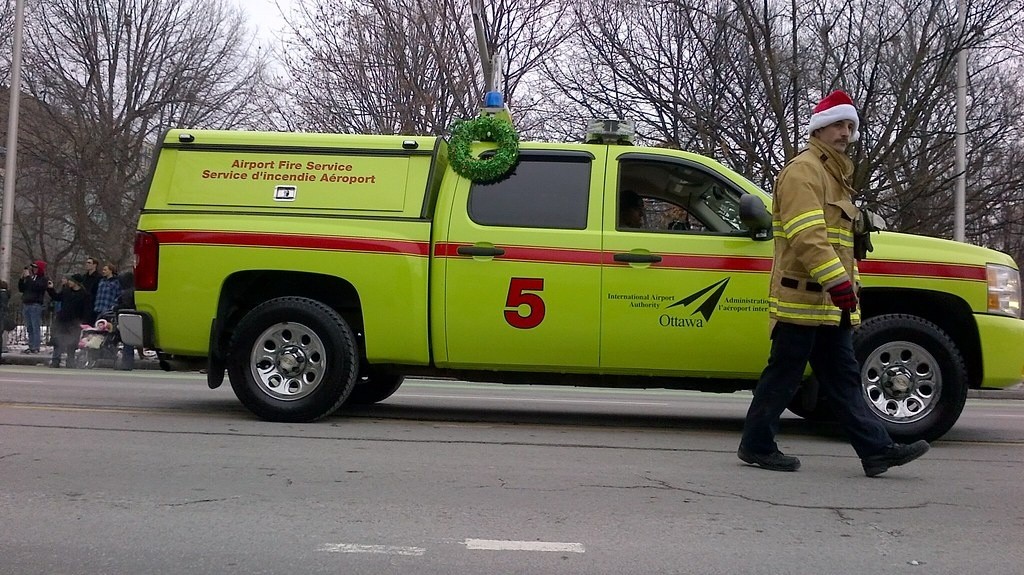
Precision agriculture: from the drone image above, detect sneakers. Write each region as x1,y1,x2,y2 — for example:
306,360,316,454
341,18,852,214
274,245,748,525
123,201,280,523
737,448,801,471
861,440,931,476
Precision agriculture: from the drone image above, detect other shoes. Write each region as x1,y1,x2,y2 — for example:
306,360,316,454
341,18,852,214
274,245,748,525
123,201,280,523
24,349,40,353
115,366,132,371
49,362,59,368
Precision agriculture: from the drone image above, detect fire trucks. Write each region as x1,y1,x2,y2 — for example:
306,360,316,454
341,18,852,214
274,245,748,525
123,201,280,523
118,94,1024,452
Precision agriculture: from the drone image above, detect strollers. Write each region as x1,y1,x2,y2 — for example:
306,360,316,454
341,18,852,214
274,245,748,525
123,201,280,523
74,311,117,371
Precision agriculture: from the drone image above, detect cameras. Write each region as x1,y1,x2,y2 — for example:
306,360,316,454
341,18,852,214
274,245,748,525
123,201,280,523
48,281,52,285
24,266,28,270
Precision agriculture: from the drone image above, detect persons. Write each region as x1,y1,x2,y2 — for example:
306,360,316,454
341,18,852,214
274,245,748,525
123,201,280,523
620,190,644,228
119,272,150,360
18,261,47,354
81,257,102,297
46,273,73,346
47,273,89,368
0,279,17,365
93,265,121,324
736,90,931,478
81,319,108,344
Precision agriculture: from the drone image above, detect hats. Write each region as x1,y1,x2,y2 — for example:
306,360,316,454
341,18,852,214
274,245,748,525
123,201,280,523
808,90,860,143
69,273,82,284
30,263,38,268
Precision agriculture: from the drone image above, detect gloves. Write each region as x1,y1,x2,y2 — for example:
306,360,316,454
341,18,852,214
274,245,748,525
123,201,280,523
829,280,858,313
